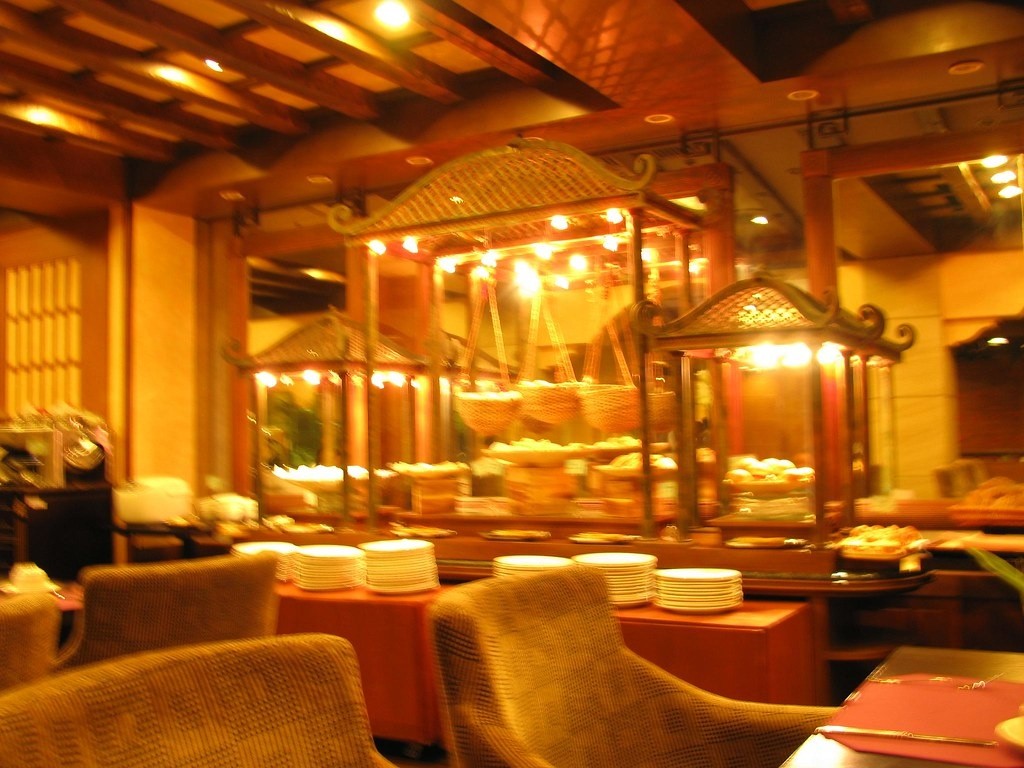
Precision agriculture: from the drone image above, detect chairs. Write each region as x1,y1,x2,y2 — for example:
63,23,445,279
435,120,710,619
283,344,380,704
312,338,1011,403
0,554,400,768
429,564,844,768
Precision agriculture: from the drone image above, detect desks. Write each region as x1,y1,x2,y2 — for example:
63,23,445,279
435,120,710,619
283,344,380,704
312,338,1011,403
271,579,452,759
778,644,1024,768
614,601,819,709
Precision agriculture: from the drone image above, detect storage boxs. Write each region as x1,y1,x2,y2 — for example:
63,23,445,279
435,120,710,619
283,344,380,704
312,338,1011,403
115,480,187,522
504,466,579,517
409,479,459,515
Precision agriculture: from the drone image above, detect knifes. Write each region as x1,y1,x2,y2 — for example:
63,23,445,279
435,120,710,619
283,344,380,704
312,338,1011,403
812,725,999,749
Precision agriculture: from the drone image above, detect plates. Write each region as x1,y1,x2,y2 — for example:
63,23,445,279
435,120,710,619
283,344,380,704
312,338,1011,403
725,534,804,549
390,525,449,536
478,529,551,540
653,569,744,616
231,538,442,594
566,533,640,544
490,551,657,610
994,714,1024,748
